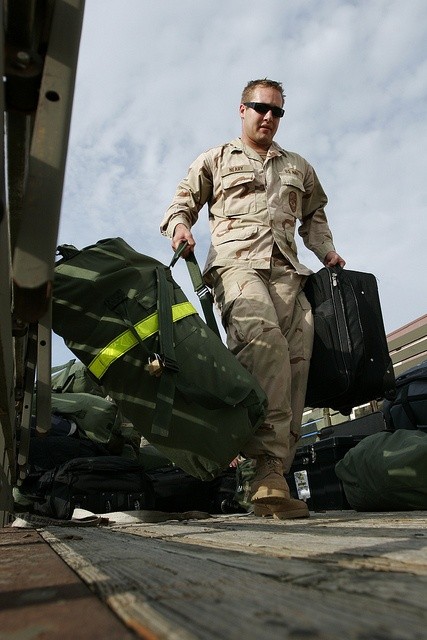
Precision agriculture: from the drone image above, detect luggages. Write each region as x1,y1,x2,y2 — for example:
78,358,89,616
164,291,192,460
143,462,240,514
18,456,145,521
303,262,398,416
315,411,389,443
284,437,391,511
18,420,136,473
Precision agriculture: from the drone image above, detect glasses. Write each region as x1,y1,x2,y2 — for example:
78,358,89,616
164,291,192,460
243,102,285,117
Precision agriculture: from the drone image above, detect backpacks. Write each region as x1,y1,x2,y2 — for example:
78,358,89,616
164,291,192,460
219,458,258,513
51,359,109,398
333,428,427,512
32,392,124,446
384,359,427,431
51,237,268,482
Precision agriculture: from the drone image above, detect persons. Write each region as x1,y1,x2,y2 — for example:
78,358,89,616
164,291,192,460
158,78,346,519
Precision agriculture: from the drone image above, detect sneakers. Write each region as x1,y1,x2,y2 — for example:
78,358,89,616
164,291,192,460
254,498,310,520
246,456,290,504
